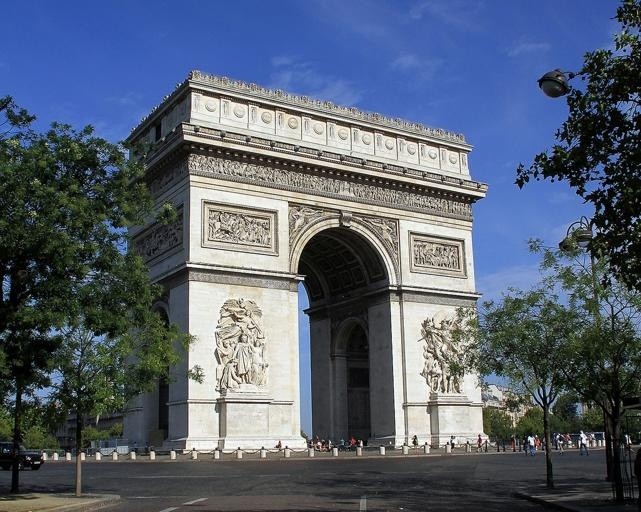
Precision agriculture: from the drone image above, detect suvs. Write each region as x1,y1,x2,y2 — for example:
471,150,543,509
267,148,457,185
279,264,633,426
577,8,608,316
0,442,44,470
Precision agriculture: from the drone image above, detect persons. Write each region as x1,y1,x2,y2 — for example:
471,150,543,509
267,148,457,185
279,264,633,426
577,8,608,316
552,433,571,454
292,206,319,237
216,298,269,391
370,218,395,251
278,440,282,450
579,431,594,454
625,434,632,450
209,212,271,246
511,435,515,448
523,435,546,454
144,441,150,455
414,243,460,269
477,434,483,452
418,317,468,394
414,435,418,454
309,436,364,452
134,441,140,454
450,436,455,452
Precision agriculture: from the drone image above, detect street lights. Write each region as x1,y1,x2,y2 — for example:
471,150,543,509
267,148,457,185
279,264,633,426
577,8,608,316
559,215,611,481
